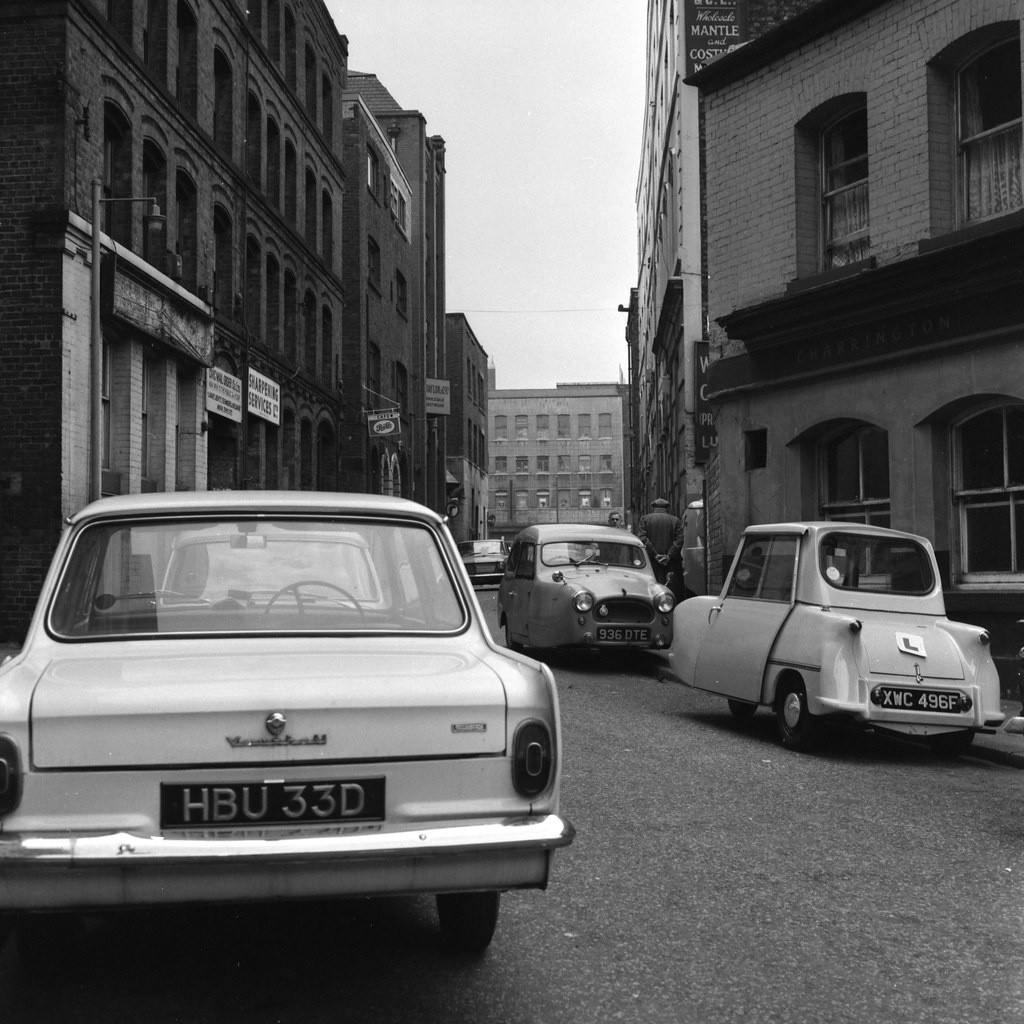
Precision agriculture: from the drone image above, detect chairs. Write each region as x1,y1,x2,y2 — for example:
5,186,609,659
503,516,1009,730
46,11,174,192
517,561,534,578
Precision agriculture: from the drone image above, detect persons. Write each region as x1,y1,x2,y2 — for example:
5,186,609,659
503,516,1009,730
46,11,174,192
608,511,622,527
639,498,684,605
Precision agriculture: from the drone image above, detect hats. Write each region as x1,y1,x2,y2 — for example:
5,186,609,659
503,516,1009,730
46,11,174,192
651,498,670,508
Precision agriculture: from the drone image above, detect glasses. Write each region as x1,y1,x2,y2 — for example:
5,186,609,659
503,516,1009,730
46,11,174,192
611,518,619,521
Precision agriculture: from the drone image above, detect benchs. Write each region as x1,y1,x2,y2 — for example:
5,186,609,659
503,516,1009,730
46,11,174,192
88,602,422,633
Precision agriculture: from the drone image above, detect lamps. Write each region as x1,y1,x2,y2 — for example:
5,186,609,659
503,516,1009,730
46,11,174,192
487,515,496,528
446,497,459,518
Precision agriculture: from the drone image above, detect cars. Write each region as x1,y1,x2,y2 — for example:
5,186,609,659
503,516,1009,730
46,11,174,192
0,489,576,952
669,521,1006,757
496,523,677,660
455,539,511,585
156,530,385,606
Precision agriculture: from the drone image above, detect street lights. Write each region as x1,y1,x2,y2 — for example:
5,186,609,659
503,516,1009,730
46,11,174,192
89,177,168,503
411,412,440,501
